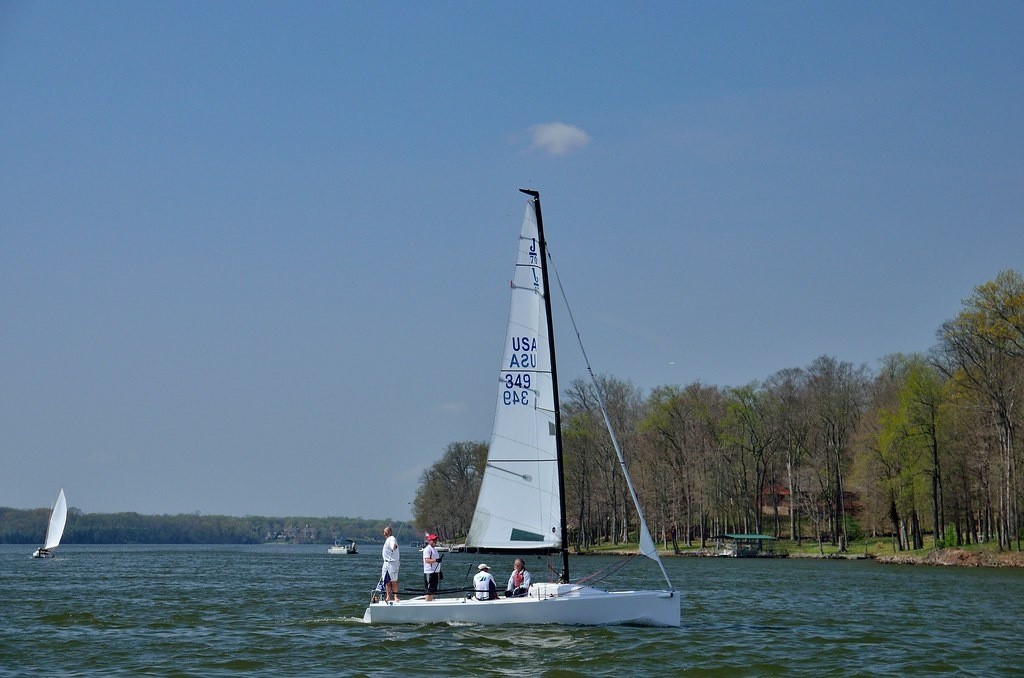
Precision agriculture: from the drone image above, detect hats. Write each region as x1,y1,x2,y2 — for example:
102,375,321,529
428,534,438,542
478,564,491,570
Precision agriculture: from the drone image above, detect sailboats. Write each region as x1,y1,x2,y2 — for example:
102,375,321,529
32,487,68,558
360,189,682,629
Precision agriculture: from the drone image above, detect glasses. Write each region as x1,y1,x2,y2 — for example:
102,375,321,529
383,530,385,533
431,538,438,542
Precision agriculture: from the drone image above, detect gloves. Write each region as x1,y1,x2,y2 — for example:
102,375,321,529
436,557,443,563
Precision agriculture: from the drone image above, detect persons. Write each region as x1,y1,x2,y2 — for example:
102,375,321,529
504,559,531,598
382,527,400,603
423,535,442,601
473,563,498,601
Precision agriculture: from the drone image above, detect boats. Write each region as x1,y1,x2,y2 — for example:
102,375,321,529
418,546,449,552
710,534,789,558
327,539,359,555
